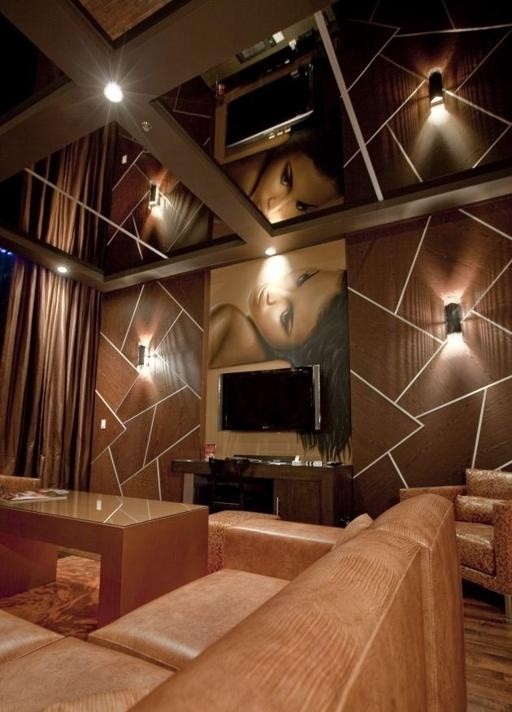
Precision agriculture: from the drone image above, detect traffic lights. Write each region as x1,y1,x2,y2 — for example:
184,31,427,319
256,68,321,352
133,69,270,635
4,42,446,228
331,512,375,551
454,494,508,526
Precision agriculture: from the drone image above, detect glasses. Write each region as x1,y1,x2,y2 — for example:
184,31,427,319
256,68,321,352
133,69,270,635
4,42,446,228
148,180,161,215
426,67,446,113
138,342,149,374
444,298,464,343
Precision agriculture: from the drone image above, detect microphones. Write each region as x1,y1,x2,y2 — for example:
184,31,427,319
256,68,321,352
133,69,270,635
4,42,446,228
209,509,281,574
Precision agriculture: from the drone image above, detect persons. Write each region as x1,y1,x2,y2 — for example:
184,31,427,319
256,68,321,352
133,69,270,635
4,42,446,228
209,268,351,463
213,112,345,223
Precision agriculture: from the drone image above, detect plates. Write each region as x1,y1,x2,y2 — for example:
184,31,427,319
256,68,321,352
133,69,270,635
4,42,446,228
170,459,355,527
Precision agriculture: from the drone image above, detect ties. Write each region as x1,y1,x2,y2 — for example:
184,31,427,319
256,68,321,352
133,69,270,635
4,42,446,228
225,63,313,150
218,365,321,432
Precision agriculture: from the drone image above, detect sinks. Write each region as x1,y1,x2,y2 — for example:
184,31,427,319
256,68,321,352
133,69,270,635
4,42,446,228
400,469,512,619
1,492,467,711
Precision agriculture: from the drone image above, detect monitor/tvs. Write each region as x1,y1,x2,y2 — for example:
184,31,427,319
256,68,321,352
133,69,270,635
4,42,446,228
0,488,69,504
